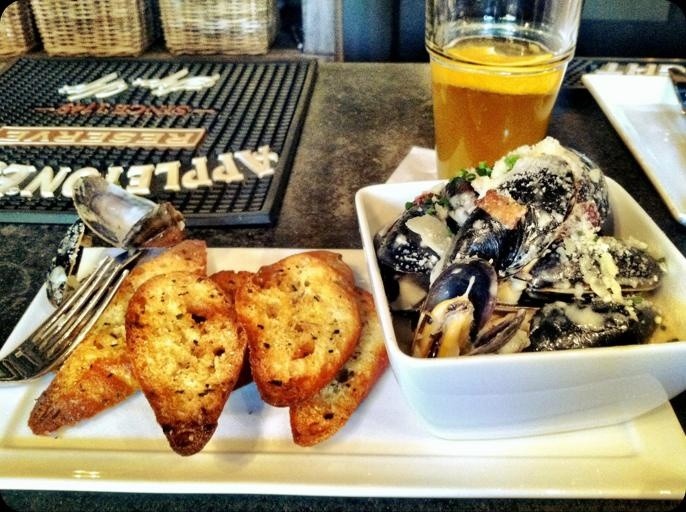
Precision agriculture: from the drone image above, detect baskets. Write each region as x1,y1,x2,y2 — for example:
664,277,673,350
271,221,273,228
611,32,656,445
29,0,160,58
0,0,40,54
157,0,279,56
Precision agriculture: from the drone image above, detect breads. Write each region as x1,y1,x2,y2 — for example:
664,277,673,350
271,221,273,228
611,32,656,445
125,272,248,456
236,251,361,407
290,286,389,446
210,270,257,390
28,240,207,435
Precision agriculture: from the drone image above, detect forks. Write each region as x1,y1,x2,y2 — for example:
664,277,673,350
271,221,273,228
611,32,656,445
1,258,129,381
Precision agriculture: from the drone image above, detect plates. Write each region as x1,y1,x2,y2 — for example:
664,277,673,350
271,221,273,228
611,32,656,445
1,240,686,502
584,72,684,223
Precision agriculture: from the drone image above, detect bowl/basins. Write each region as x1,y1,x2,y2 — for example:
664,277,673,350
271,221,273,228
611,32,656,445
356,170,685,439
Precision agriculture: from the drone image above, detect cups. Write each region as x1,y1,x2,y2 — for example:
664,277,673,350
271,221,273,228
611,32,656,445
424,0,582,181
